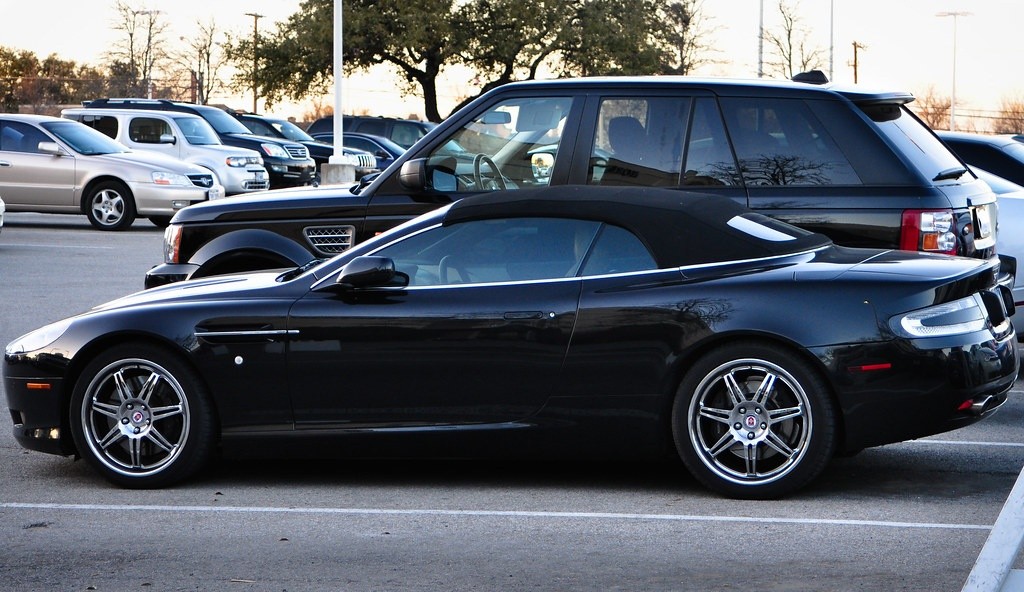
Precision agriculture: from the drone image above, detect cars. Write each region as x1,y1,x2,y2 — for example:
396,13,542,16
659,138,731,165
4,186,1023,504
306,132,407,178
0,111,225,232
929,126,1019,348
229,113,378,183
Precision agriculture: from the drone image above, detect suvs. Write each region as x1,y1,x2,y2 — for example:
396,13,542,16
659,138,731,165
80,99,320,188
143,70,1007,295
60,108,272,195
312,114,483,169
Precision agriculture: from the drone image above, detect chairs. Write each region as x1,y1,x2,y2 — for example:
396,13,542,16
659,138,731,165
138,125,152,140
565,226,602,278
598,116,645,184
